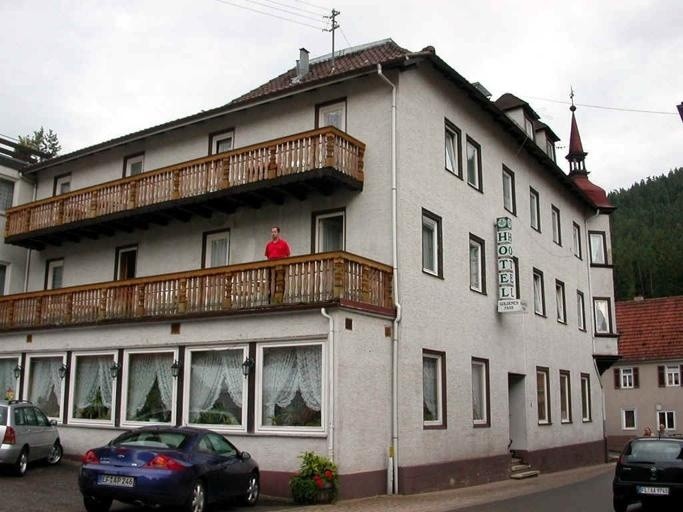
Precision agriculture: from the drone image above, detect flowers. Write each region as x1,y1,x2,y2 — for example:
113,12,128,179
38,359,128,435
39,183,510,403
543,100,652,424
289,451,339,504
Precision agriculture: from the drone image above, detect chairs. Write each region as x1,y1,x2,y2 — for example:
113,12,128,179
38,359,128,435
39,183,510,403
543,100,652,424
144,436,162,442
27,416,41,425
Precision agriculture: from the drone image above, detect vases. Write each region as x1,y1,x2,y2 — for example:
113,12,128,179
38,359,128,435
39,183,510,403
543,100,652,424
291,478,335,504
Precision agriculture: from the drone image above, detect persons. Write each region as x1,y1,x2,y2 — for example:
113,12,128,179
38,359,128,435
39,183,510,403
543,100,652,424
643,423,669,437
265,224,290,304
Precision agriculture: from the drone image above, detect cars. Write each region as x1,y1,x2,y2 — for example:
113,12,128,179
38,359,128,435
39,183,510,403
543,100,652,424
133,409,239,425
1,400,63,476
78,426,260,512
73,403,111,420
612,433,683,512
